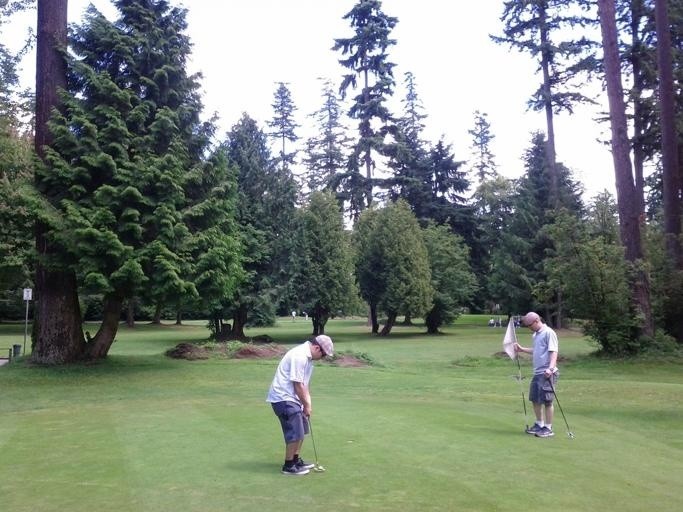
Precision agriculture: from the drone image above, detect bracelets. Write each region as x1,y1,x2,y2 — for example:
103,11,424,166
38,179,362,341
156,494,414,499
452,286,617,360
548,367,555,372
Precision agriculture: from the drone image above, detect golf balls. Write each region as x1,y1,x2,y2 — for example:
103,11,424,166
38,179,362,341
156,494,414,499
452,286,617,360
320,466,323,468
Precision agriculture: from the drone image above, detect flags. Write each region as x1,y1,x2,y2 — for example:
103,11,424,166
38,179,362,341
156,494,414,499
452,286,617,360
501,318,517,360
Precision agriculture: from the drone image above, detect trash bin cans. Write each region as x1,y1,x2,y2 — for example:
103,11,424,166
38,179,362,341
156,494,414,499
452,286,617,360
12,344,21,356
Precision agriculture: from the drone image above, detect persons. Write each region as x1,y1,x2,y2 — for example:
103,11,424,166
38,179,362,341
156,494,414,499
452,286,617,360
302,311,309,320
290,310,296,321
265,333,336,478
511,310,559,439
487,317,520,329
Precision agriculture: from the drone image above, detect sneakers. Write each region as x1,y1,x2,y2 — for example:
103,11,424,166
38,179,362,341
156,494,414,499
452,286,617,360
534,427,554,437
293,458,314,469
281,465,310,475
525,423,542,434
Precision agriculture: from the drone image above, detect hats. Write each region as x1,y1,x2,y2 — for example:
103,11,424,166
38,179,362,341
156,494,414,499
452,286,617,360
315,334,334,357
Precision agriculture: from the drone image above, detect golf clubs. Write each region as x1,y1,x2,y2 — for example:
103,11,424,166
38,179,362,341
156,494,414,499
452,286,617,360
543,370,576,440
309,419,326,472
